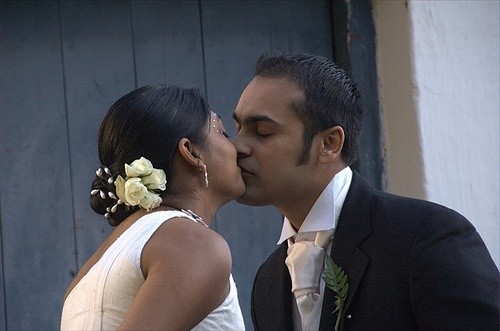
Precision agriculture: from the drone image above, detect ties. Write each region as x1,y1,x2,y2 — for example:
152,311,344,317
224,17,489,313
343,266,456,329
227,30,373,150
285,184,350,331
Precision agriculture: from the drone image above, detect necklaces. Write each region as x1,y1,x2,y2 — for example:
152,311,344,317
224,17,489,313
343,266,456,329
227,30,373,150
161,202,210,228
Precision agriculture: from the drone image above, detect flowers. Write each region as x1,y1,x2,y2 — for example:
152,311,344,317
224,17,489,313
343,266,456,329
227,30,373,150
114,157,167,212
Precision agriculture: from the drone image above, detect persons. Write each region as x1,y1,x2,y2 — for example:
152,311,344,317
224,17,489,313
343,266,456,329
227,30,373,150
230,49,500,331
60,82,247,331
